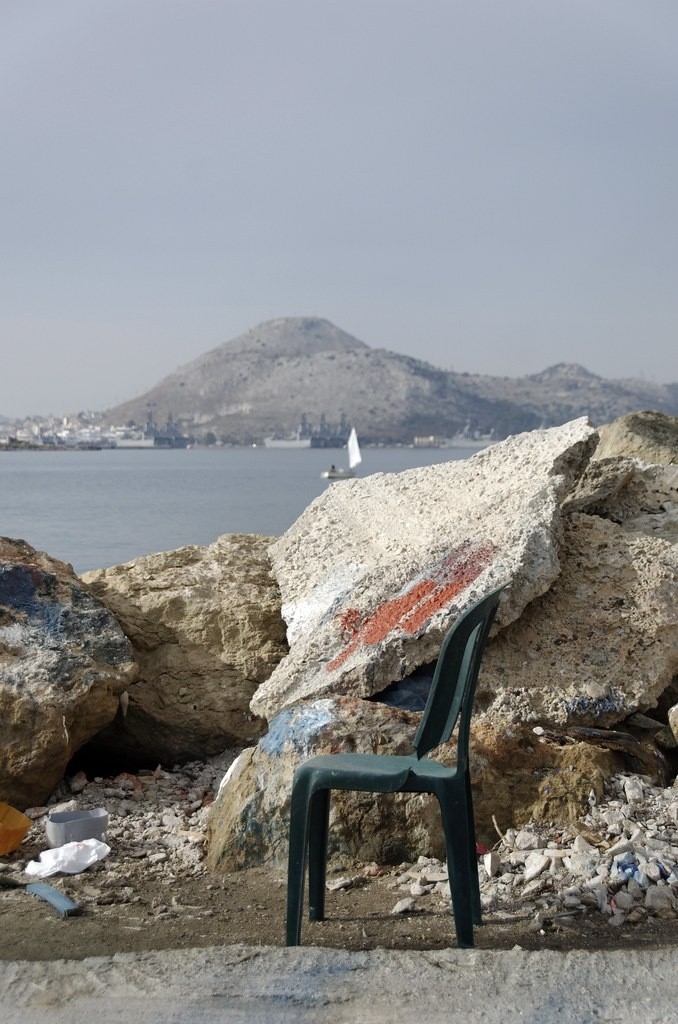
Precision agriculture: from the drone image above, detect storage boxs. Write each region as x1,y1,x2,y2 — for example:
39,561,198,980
0,801,34,857
44,807,109,850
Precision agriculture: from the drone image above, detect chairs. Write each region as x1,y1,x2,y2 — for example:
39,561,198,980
285,578,511,948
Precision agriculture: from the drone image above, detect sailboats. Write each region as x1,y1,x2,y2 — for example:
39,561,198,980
320,427,363,478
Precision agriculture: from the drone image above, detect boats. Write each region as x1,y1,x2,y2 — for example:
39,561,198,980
262,420,338,448
116,436,171,448
439,417,501,449
